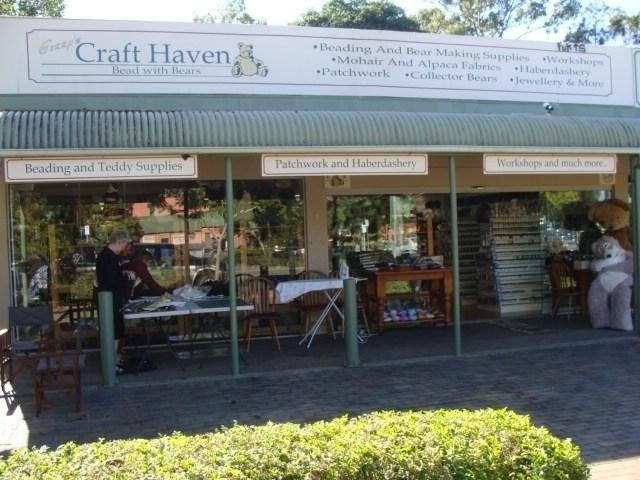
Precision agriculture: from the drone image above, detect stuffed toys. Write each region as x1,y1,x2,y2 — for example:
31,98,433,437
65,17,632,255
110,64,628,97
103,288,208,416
587,234,634,332
587,196,632,250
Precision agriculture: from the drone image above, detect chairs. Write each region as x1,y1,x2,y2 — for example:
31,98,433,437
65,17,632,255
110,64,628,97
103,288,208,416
0,298,99,417
233,269,372,353
544,263,580,320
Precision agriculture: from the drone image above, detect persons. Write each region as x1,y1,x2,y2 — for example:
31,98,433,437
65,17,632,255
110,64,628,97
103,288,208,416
580,220,604,254
118,243,133,266
114,251,174,354
96,230,134,375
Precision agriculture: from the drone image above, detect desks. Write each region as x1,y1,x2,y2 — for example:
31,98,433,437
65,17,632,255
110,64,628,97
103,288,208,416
566,264,597,320
363,264,453,337
121,295,255,375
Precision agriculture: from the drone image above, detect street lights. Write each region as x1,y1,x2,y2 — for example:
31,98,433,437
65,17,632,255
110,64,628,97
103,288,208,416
381,214,387,236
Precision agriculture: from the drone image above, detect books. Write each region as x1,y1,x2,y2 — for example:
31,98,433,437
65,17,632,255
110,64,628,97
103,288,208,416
359,252,386,272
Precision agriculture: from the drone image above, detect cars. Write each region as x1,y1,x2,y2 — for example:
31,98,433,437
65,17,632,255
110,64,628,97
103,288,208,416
539,218,578,244
11,240,236,304
344,235,445,276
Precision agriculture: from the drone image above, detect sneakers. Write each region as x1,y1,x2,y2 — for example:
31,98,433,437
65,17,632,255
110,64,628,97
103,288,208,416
116,359,127,373
